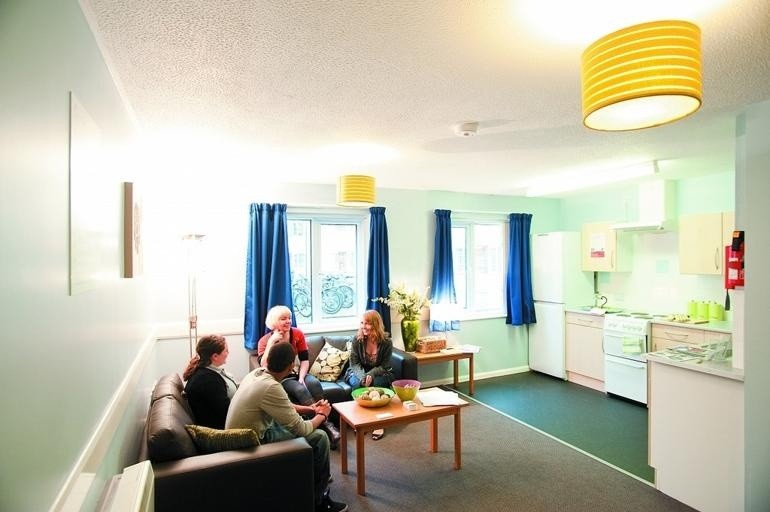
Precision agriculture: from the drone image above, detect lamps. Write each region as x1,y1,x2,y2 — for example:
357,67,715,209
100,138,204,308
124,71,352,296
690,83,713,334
581,20,704,131
336,174,376,208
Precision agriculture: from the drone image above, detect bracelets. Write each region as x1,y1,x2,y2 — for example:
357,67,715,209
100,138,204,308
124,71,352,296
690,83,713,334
317,412,328,423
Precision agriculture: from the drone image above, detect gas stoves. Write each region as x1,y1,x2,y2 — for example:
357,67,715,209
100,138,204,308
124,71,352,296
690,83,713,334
604,311,674,336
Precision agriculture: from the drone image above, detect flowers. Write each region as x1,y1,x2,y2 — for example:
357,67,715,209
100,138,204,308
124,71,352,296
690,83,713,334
369,286,434,320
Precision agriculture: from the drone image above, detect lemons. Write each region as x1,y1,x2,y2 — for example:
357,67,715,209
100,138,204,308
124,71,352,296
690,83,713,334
376,389,385,396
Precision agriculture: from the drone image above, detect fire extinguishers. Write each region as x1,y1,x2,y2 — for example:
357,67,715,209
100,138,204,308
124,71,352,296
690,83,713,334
728,231,744,290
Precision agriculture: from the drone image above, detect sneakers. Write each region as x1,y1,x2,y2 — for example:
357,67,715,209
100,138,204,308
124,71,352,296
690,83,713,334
316,489,348,512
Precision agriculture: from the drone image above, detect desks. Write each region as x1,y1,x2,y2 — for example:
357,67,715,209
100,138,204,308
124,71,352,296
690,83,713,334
332,386,471,496
393,347,474,395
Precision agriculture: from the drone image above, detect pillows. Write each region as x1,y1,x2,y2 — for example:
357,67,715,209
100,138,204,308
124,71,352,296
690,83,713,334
184,422,261,454
309,341,353,382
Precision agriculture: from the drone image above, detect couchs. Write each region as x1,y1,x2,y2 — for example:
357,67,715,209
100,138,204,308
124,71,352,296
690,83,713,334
136,369,316,512
249,332,417,406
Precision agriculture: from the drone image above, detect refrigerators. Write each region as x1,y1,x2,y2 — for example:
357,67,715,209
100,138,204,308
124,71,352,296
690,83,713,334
527,229,599,380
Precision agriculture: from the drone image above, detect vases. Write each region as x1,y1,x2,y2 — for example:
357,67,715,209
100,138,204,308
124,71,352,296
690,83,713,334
399,318,424,352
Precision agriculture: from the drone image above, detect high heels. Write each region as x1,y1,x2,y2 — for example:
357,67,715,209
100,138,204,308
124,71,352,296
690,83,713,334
325,422,340,450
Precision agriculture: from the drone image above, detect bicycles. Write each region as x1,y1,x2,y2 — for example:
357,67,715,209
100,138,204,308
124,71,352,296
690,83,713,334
291,269,355,318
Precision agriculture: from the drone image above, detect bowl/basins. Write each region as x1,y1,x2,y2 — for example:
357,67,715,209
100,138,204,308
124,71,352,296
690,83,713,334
391,379,421,402
351,387,395,408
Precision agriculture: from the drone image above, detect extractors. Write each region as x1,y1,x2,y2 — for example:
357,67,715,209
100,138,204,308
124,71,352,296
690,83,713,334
610,179,672,233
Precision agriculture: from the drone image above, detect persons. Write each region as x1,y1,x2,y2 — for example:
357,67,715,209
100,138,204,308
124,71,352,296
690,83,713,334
225,342,348,512
343,310,393,441
183,334,239,430
258,305,341,450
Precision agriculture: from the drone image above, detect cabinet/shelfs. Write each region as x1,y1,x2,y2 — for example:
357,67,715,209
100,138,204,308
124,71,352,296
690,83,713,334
648,339,745,512
648,322,736,470
563,304,608,394
677,210,735,276
525,299,569,383
605,310,676,407
